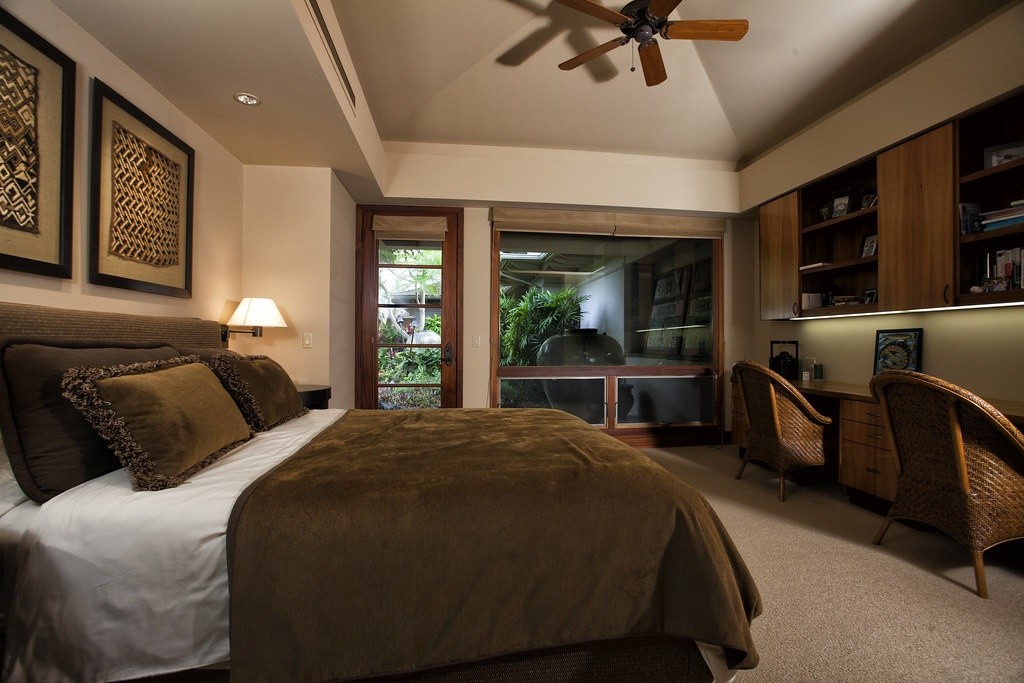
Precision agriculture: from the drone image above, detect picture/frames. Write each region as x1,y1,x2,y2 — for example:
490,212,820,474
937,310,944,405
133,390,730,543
771,340,799,380
828,191,850,219
857,188,877,210
984,141,1024,170
967,212,985,233
858,229,878,259
0,9,76,281
873,327,923,376
861,287,878,304
639,256,716,361
86,76,197,298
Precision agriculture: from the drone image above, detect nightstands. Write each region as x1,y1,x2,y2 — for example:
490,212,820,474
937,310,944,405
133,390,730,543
294,384,332,409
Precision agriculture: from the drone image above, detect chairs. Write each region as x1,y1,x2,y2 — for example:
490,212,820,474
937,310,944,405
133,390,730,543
731,360,840,502
869,369,1023,598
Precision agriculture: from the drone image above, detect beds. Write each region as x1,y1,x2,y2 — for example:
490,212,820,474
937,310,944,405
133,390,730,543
0,302,764,683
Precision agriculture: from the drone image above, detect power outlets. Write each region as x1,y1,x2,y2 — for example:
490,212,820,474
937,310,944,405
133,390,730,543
804,357,816,372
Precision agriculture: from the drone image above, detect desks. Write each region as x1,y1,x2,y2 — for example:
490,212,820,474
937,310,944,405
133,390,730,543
730,376,1024,530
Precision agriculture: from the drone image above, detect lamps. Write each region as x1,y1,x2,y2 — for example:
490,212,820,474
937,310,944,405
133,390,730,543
220,298,287,343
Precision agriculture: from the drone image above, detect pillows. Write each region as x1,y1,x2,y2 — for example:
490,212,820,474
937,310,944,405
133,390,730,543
59,355,256,495
178,345,242,366
208,353,313,433
1,338,182,506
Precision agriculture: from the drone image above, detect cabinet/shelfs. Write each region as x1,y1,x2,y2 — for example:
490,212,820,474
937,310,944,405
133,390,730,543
756,86,1024,323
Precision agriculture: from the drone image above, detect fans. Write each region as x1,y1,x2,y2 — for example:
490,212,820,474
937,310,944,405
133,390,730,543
557,1,750,88
